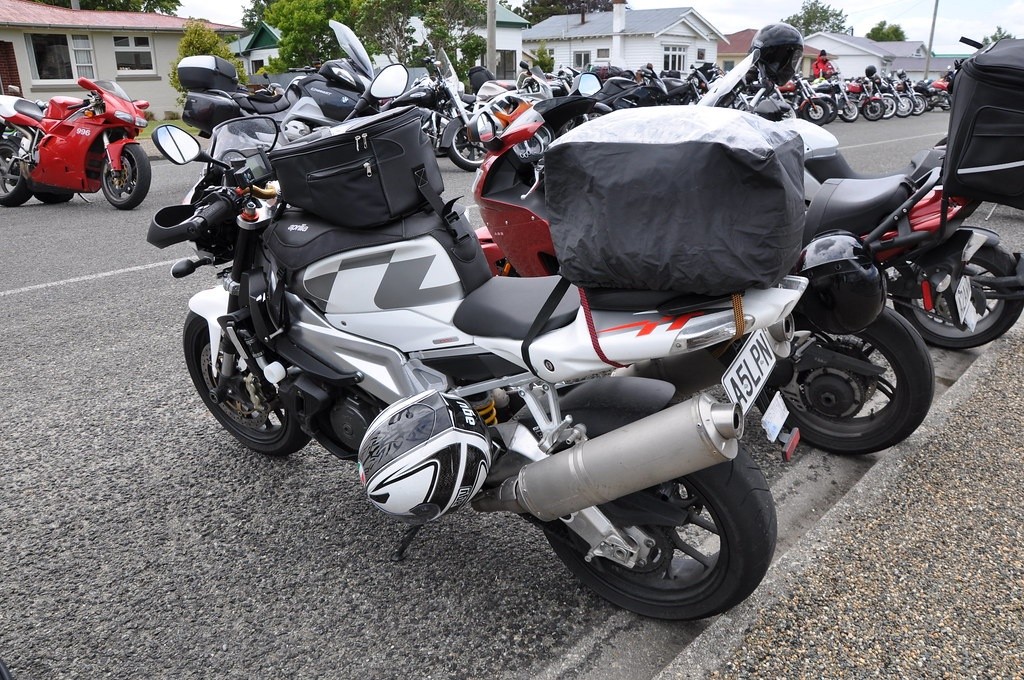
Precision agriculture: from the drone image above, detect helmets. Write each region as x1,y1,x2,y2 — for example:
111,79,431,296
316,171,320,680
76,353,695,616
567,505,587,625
865,65,877,78
356,388,493,526
794,229,889,337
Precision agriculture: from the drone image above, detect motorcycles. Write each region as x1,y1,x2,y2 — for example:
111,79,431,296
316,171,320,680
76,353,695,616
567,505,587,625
568,21,1024,351
0,77,152,211
145,63,777,621
407,15,937,463
177,54,951,172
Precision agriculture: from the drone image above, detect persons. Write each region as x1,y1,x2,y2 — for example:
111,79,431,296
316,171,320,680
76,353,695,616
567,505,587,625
811,50,834,79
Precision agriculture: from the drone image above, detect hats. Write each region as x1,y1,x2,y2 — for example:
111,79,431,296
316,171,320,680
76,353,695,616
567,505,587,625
819,49,827,57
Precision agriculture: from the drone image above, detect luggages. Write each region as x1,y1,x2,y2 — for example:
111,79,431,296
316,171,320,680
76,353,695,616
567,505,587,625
938,37,1023,211
266,103,446,231
